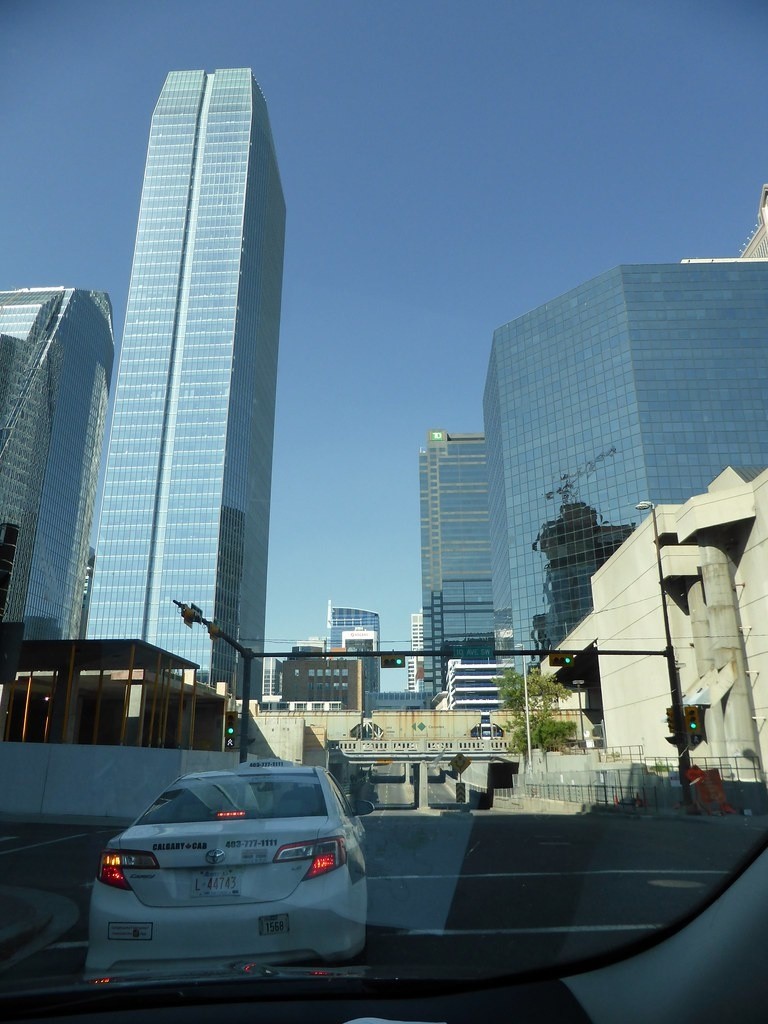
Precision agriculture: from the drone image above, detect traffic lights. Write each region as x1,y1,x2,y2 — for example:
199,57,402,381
242,736,256,748
184,607,198,621
224,711,237,739
667,708,675,732
209,622,223,638
548,654,575,667
380,656,405,668
685,706,701,735
665,735,678,747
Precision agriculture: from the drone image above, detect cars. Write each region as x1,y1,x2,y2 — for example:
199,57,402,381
84,766,376,980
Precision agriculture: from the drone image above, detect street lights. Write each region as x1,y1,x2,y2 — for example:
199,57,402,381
634,501,702,817
